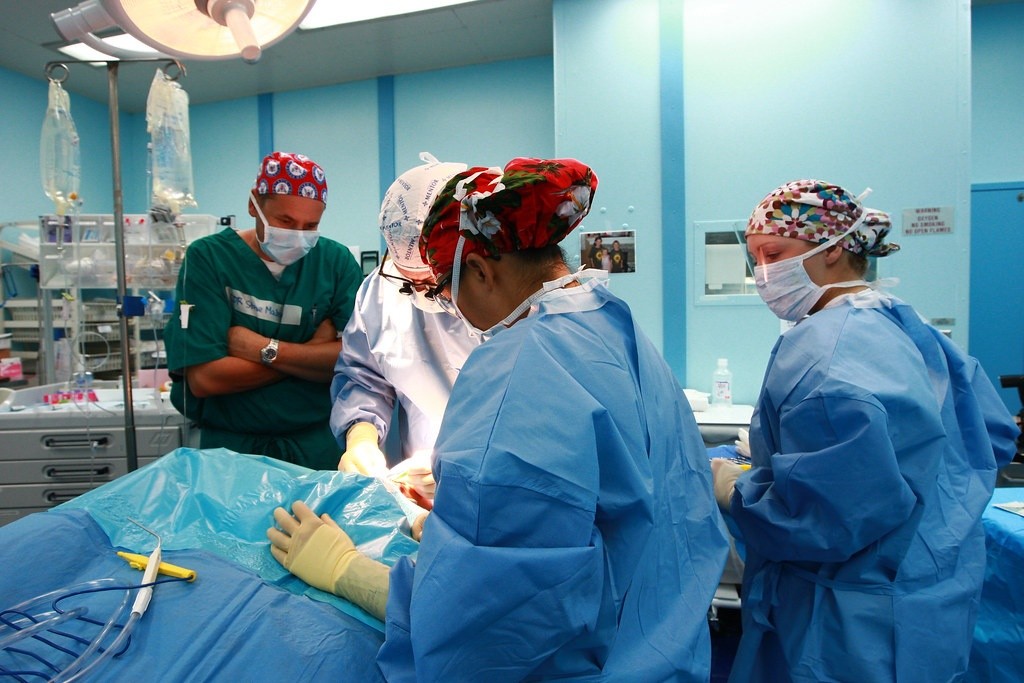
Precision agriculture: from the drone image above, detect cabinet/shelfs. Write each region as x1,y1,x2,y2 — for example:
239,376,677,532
0,380,201,527
0,223,165,390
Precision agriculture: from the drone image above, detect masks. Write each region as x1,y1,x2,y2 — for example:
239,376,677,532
450,259,510,344
409,286,446,314
255,196,323,266
754,237,836,323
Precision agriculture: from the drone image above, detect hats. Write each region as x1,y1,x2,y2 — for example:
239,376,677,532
419,160,597,283
744,180,899,256
378,154,469,269
251,148,329,203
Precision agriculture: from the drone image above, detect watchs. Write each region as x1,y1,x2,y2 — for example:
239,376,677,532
261,338,280,367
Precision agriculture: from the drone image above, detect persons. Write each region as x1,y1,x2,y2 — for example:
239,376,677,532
711,179,1019,683
586,238,628,273
328,152,491,500
164,150,365,472
265,158,730,683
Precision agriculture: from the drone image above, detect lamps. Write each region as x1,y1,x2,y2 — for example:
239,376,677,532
49,0,316,65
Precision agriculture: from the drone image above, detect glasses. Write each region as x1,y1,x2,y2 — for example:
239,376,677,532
432,253,501,315
379,246,463,302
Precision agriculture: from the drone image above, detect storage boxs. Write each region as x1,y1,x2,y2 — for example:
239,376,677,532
38,213,217,290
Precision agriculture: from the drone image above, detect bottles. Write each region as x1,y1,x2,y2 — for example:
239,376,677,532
711,358,731,409
